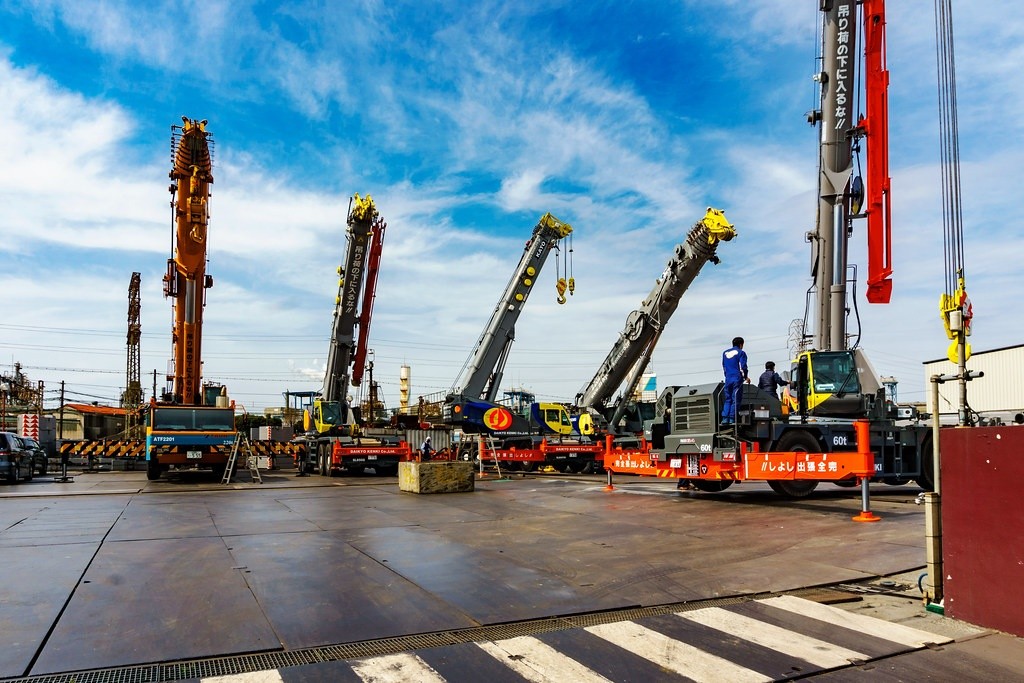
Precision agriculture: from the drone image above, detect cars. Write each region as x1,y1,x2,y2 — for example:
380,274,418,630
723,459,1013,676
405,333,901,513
21,438,48,477
0,432,36,484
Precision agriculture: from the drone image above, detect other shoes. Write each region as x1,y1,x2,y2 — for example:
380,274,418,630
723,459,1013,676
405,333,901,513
679,487,689,490
688,486,694,489
729,419,734,423
721,418,728,424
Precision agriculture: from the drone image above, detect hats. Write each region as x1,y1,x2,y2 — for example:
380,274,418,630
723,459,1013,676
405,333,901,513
427,437,431,440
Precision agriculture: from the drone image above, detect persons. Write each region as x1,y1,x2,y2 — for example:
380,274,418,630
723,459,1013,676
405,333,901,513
757,360,790,400
720,336,751,424
421,437,436,460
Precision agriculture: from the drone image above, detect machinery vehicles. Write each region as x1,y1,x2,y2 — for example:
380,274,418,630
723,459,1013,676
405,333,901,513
604,0,973,499
138,115,247,481
294,192,413,476
442,205,738,476
